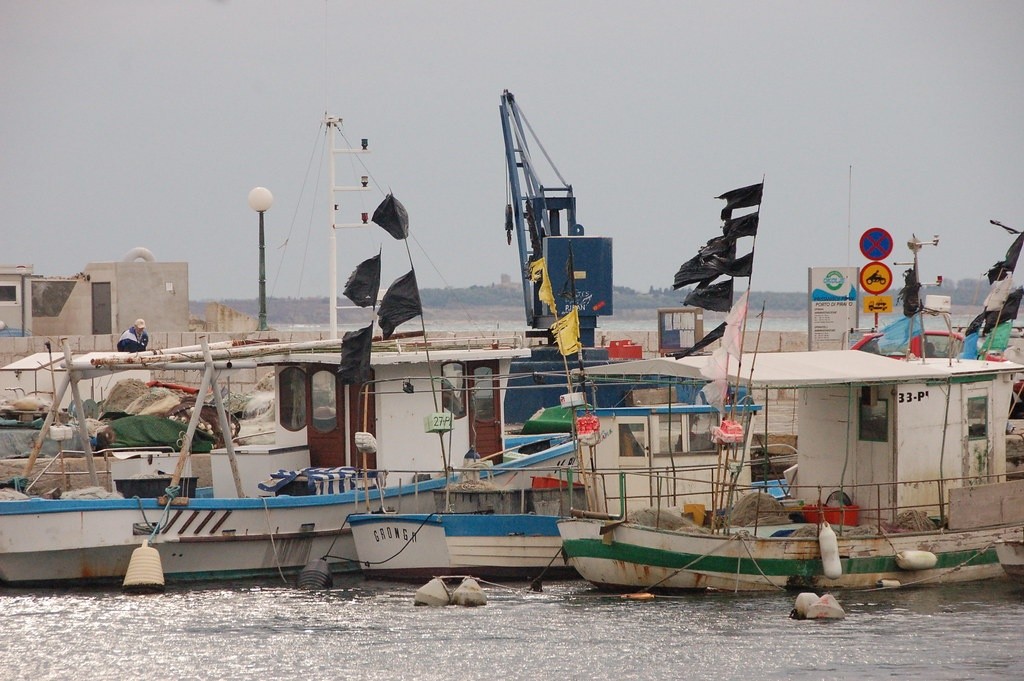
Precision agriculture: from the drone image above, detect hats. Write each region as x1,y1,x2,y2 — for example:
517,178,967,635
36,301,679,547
135,318,145,328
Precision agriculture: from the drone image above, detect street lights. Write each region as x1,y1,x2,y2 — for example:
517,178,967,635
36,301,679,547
249,187,273,328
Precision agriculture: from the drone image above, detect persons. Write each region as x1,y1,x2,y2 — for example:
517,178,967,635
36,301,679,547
116,318,149,352
1008,379,1024,419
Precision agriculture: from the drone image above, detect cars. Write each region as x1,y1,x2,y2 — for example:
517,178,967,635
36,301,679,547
852,331,1024,420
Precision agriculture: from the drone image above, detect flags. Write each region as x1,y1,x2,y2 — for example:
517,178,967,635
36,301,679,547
880,228,1024,366
668,183,763,445
378,271,420,341
506,200,582,355
343,252,382,311
337,325,371,387
441,378,462,417
373,195,410,239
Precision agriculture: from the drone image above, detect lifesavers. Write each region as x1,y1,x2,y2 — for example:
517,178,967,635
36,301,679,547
122,246,156,262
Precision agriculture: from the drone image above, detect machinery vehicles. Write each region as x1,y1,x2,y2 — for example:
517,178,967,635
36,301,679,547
498,88,762,428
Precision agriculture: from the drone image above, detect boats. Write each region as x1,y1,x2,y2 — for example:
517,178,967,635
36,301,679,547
343,402,792,585
555,351,1024,594
0,116,531,588
